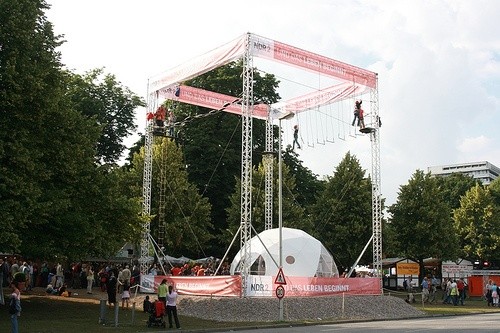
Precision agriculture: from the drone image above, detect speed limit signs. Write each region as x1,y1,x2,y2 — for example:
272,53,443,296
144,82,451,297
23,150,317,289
276,285,285,299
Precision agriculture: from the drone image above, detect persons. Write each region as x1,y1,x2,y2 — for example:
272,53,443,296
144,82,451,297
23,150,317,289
156,298,167,327
168,109,176,137
121,280,131,310
402,274,470,306
8,281,21,316
352,99,362,127
107,281,118,308
491,282,499,307
486,279,494,306
0,258,231,295
142,295,152,327
291,125,301,150
158,279,169,309
155,104,166,133
7,289,21,333
165,285,181,330
342,269,385,278
359,106,365,129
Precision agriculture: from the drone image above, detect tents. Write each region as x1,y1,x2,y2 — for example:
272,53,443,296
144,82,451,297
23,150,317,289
345,265,381,272
159,255,222,264
